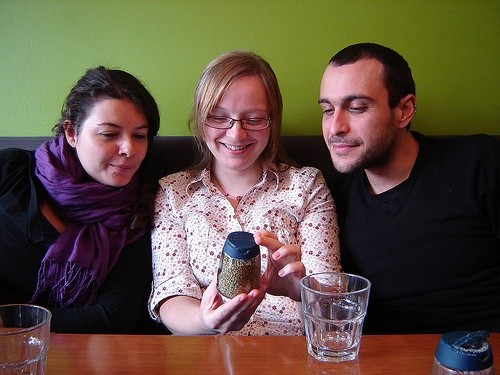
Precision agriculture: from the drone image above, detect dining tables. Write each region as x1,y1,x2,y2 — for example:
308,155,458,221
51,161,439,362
0,326,500,375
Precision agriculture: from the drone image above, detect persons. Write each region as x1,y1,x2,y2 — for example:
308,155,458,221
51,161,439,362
148,51,349,335
0,65,160,334
318,43,500,335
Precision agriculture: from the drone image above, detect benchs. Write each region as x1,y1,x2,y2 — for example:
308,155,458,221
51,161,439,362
0,136,500,181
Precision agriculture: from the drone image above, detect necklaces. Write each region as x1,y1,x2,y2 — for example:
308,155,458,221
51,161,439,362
211,172,263,200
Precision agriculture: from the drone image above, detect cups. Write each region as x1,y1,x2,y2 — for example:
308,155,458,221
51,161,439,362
0,304,53,375
299,271,371,362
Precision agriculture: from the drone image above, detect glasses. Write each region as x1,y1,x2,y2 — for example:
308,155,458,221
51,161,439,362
204,115,271,131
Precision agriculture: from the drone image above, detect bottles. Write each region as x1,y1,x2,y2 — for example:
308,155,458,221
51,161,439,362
431,330,495,375
215,232,261,302
